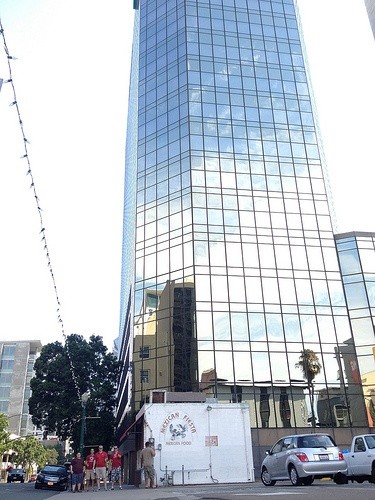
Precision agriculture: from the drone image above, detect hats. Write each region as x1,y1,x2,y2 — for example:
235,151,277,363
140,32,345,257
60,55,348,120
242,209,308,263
98,446,103,449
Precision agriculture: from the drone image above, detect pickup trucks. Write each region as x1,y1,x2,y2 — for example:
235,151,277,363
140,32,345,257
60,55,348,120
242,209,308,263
340,433,375,484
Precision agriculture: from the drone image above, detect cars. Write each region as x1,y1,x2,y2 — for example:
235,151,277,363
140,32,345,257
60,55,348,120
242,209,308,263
34,461,73,492
260,432,348,486
28,474,38,482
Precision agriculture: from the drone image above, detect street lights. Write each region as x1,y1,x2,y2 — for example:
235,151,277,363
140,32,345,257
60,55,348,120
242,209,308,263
80,390,90,457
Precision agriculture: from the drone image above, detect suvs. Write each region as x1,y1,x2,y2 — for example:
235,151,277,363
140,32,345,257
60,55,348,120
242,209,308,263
7,468,26,483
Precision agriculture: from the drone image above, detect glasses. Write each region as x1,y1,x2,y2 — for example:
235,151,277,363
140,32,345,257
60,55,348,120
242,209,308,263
114,448,117,449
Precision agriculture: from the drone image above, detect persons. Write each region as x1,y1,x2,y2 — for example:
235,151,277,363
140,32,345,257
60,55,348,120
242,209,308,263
111,446,123,490
93,445,109,491
86,448,95,492
139,442,155,488
71,452,86,493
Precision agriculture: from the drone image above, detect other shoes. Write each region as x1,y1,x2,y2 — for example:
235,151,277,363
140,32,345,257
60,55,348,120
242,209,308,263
98,488,100,491
91,489,94,492
119,486,122,490
86,490,89,492
73,490,76,493
109,487,114,491
77,490,82,493
104,488,107,491
